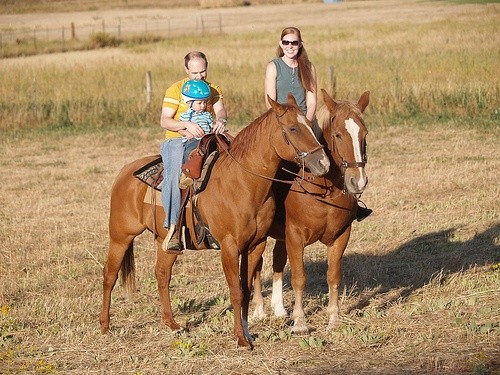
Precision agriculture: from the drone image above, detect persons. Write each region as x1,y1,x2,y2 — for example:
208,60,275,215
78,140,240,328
160,51,228,254
177,79,228,189
265,26,373,222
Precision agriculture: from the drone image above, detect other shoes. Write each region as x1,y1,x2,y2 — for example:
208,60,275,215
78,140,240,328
178,177,194,189
355,207,373,222
167,229,182,250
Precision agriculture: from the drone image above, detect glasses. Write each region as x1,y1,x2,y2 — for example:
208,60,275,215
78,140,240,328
282,40,300,46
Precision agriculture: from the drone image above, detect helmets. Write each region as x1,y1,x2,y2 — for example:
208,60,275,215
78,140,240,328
182,80,212,99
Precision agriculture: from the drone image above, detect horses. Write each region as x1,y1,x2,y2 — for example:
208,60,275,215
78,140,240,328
99,88,370,352
